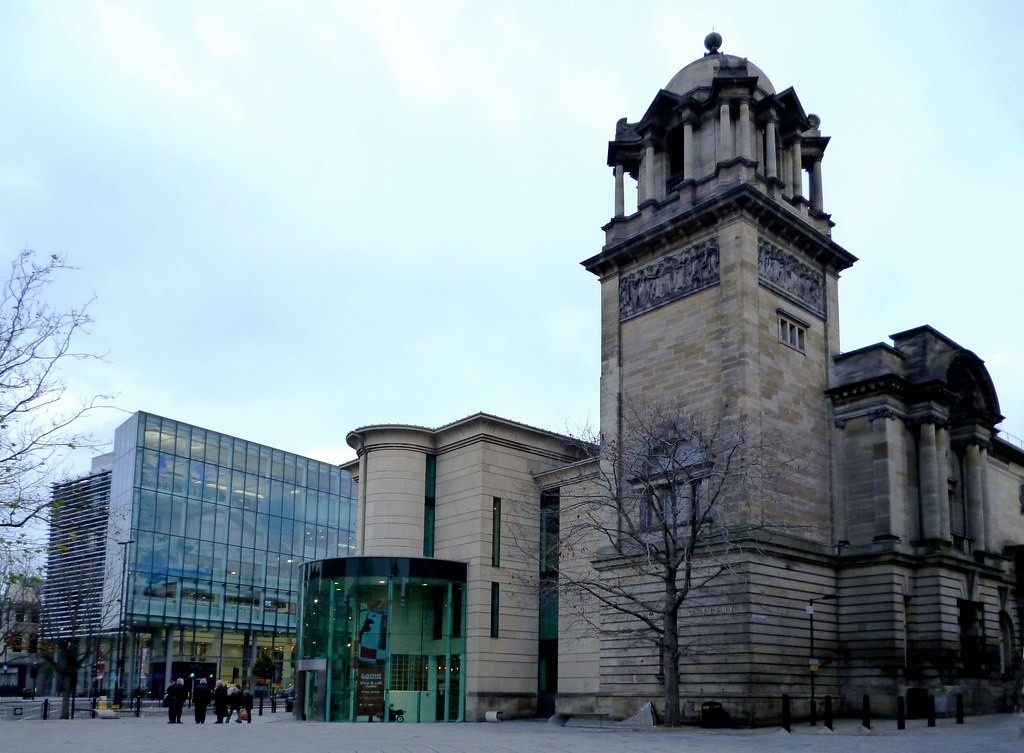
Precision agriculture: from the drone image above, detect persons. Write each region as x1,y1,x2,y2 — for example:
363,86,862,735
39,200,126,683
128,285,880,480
164,679,175,707
213,681,229,724
192,678,211,724
243,690,253,723
225,683,242,723
166,678,187,724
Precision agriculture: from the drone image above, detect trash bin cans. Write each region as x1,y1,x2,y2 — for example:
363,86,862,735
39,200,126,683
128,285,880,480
23,688,32,700
906,688,929,720
285,699,293,712
702,701,724,729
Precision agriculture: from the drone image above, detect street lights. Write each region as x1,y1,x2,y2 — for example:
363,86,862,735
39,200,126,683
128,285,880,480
808,592,841,727
113,540,137,705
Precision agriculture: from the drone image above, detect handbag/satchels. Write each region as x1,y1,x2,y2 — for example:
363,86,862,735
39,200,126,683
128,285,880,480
162,698,169,707
241,708,248,720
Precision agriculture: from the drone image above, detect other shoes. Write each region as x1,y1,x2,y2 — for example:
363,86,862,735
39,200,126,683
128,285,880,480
167,721,175,724
177,721,182,724
215,721,222,723
236,720,241,723
225,720,229,722
